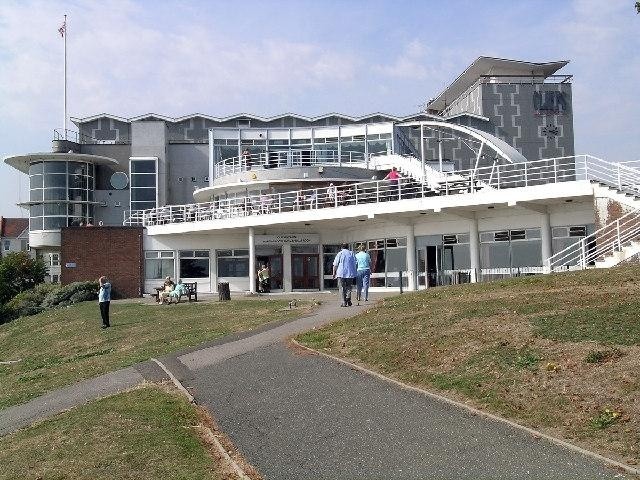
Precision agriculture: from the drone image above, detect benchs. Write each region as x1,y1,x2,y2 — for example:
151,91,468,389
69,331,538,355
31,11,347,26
155,282,198,302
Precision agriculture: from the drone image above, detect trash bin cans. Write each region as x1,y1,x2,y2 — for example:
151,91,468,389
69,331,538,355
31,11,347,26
218,282,230,302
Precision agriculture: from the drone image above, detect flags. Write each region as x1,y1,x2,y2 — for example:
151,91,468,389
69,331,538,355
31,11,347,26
58,20,66,37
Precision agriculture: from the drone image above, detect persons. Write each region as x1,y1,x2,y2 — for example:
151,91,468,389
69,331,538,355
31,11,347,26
258,265,271,292
383,167,403,198
356,244,372,302
156,276,174,302
159,281,173,305
260,193,269,214
241,147,253,166
327,183,339,206
98,276,111,329
258,267,268,292
71,219,103,227
332,243,358,307
169,279,189,304
